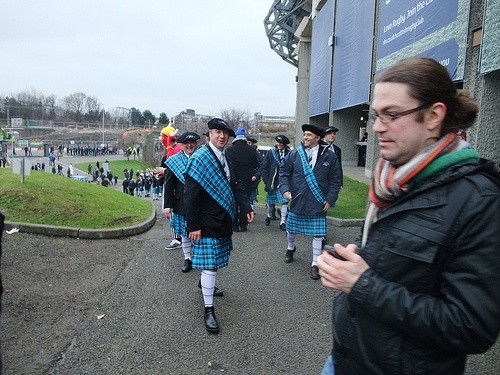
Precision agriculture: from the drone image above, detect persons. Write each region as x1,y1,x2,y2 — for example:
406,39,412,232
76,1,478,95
0,212,6,317
163,132,210,272
279,124,338,280
121,168,162,201
67,146,117,156
31,161,45,172
184,117,253,333
322,127,343,248
88,159,118,187
51,165,56,174
67,163,73,177
316,57,500,375
127,145,140,155
0,157,7,168
24,145,33,156
360,132,367,163
49,152,55,167
58,163,63,175
13,142,17,155
225,128,291,232
49,143,64,155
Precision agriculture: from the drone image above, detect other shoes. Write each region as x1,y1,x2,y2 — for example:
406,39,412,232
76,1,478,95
271,208,278,220
232,224,247,232
322,239,327,249
276,207,289,217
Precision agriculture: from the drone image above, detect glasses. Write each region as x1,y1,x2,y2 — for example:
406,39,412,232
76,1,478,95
369,100,435,124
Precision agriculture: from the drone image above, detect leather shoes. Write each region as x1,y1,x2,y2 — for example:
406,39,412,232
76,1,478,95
198,279,223,296
182,258,191,273
279,223,286,232
284,246,296,264
265,216,271,225
310,265,321,280
204,306,218,331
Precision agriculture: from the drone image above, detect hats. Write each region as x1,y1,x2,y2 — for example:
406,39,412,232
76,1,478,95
234,127,247,138
207,118,236,138
160,126,177,139
302,124,325,139
275,135,290,145
203,131,209,137
246,134,257,143
324,126,339,135
176,132,201,142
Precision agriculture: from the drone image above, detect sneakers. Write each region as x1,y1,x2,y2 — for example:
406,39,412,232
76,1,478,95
165,239,182,249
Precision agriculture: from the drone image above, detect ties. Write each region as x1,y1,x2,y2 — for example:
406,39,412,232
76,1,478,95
220,153,226,175
309,149,313,169
280,149,283,159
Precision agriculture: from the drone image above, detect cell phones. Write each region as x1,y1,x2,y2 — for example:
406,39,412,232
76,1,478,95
324,244,346,261
